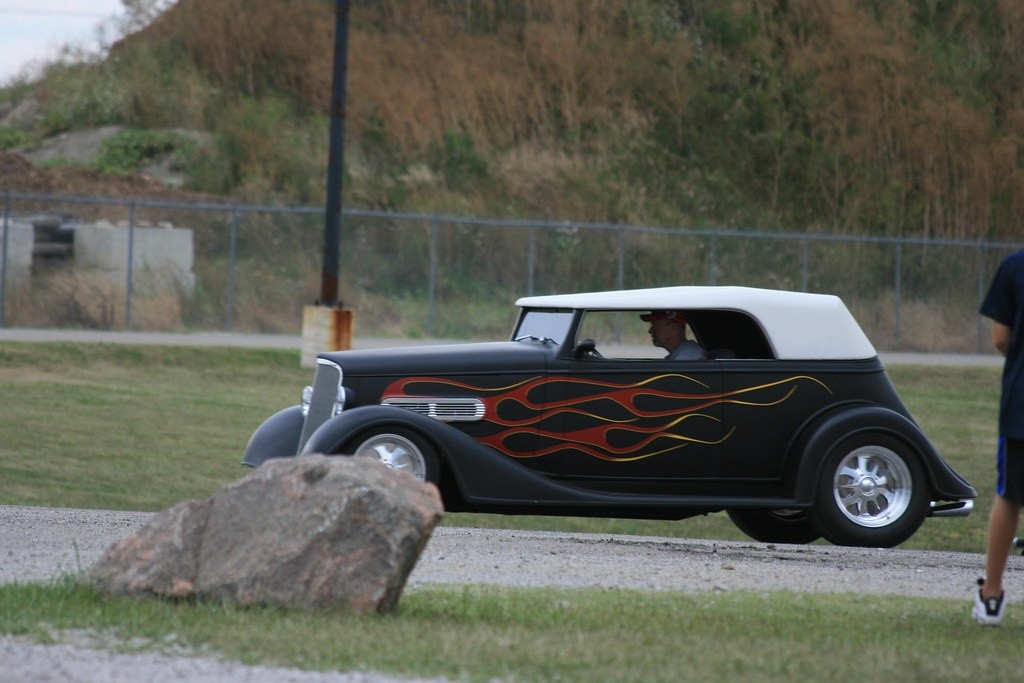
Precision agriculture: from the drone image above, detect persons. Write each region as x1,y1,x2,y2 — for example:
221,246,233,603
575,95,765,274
970,250,1023,628
639,309,705,360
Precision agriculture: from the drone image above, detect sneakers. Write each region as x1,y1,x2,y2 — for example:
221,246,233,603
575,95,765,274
972,577,1005,627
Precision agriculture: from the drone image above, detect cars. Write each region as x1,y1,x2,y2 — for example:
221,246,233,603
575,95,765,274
240,284,977,546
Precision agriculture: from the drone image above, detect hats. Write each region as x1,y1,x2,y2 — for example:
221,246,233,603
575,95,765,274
640,311,686,323
